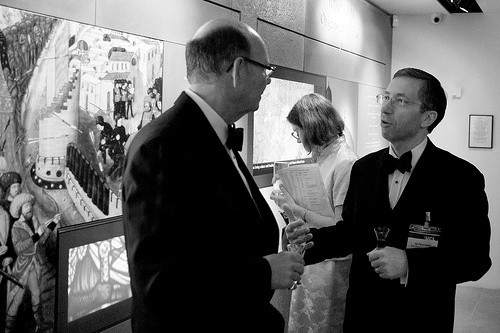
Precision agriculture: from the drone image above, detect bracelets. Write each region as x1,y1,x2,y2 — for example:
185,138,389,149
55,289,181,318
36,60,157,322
300,209,307,218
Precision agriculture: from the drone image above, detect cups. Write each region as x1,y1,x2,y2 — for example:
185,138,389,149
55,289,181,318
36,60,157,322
287,243,308,290
374,227,390,248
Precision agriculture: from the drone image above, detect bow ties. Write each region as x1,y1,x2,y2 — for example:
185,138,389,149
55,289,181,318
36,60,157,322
387,151,412,174
226,123,244,152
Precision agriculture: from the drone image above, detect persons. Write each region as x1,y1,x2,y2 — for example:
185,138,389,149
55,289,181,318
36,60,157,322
122,17,305,333
284,68,492,333
270,93,361,333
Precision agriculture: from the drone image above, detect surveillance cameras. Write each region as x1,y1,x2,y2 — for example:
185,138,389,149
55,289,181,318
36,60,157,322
431,12,442,24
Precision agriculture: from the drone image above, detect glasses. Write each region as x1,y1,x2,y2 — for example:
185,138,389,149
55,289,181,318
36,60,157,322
375,94,428,108
291,124,303,139
226,56,276,80
